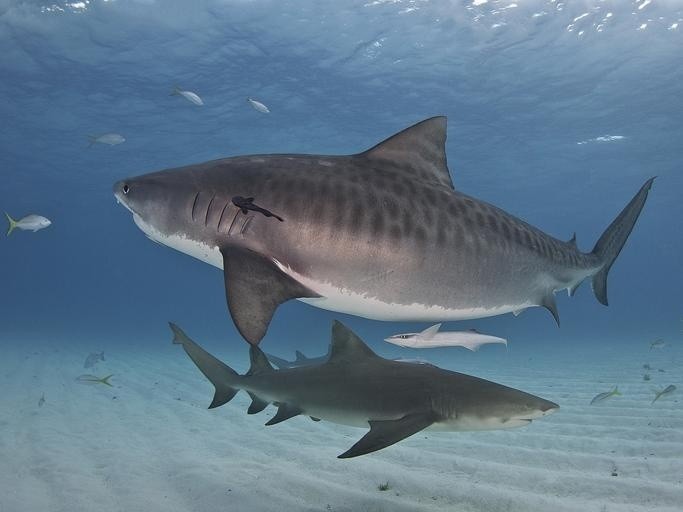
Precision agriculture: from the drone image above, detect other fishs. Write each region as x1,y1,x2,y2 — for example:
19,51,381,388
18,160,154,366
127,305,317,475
231,195,284,223
577,133,628,147
